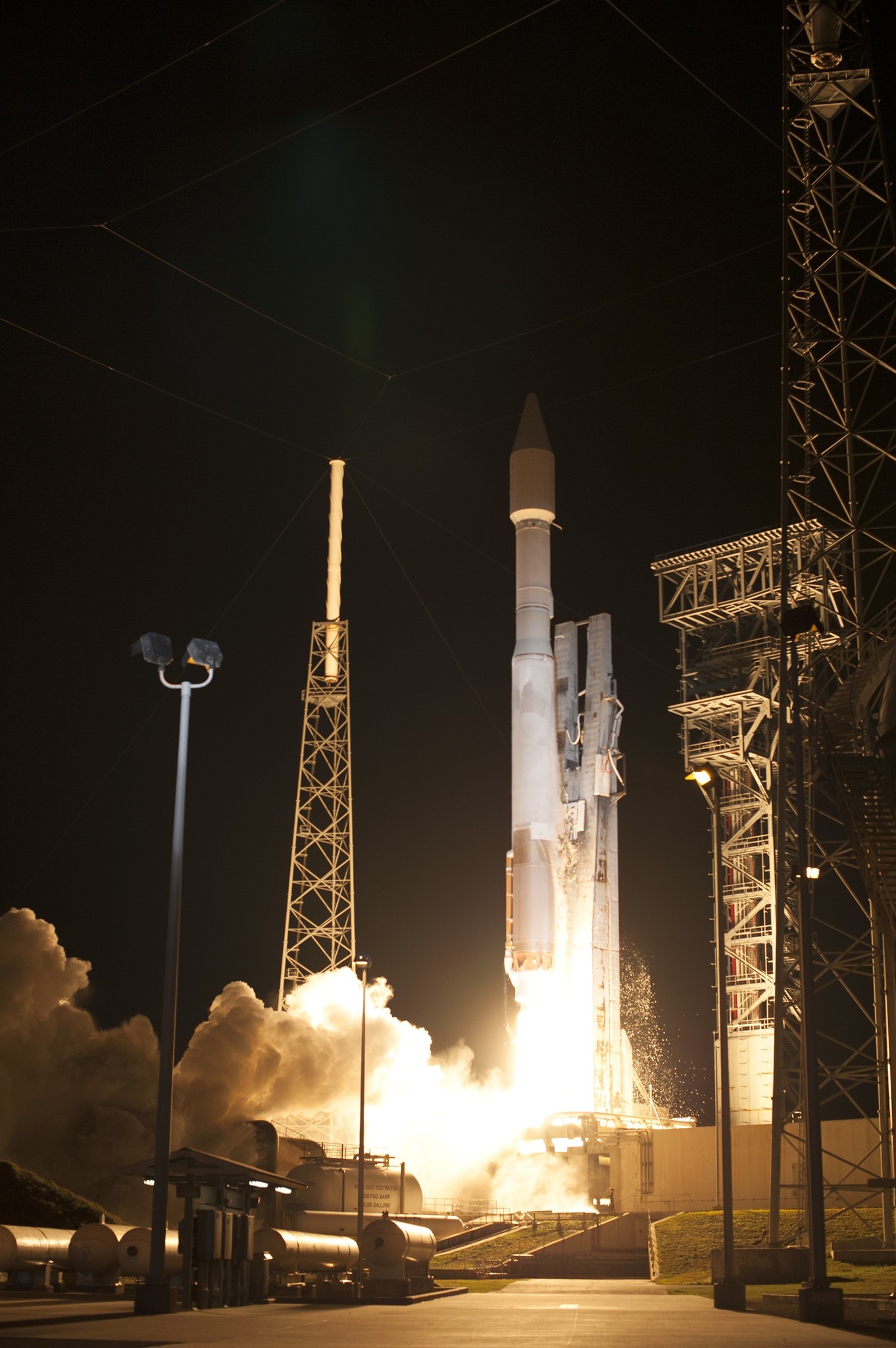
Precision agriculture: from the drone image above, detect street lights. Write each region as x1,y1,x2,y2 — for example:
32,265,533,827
352,952,368,1282
750,864,827,1287
684,760,736,1280
135,630,224,1286
